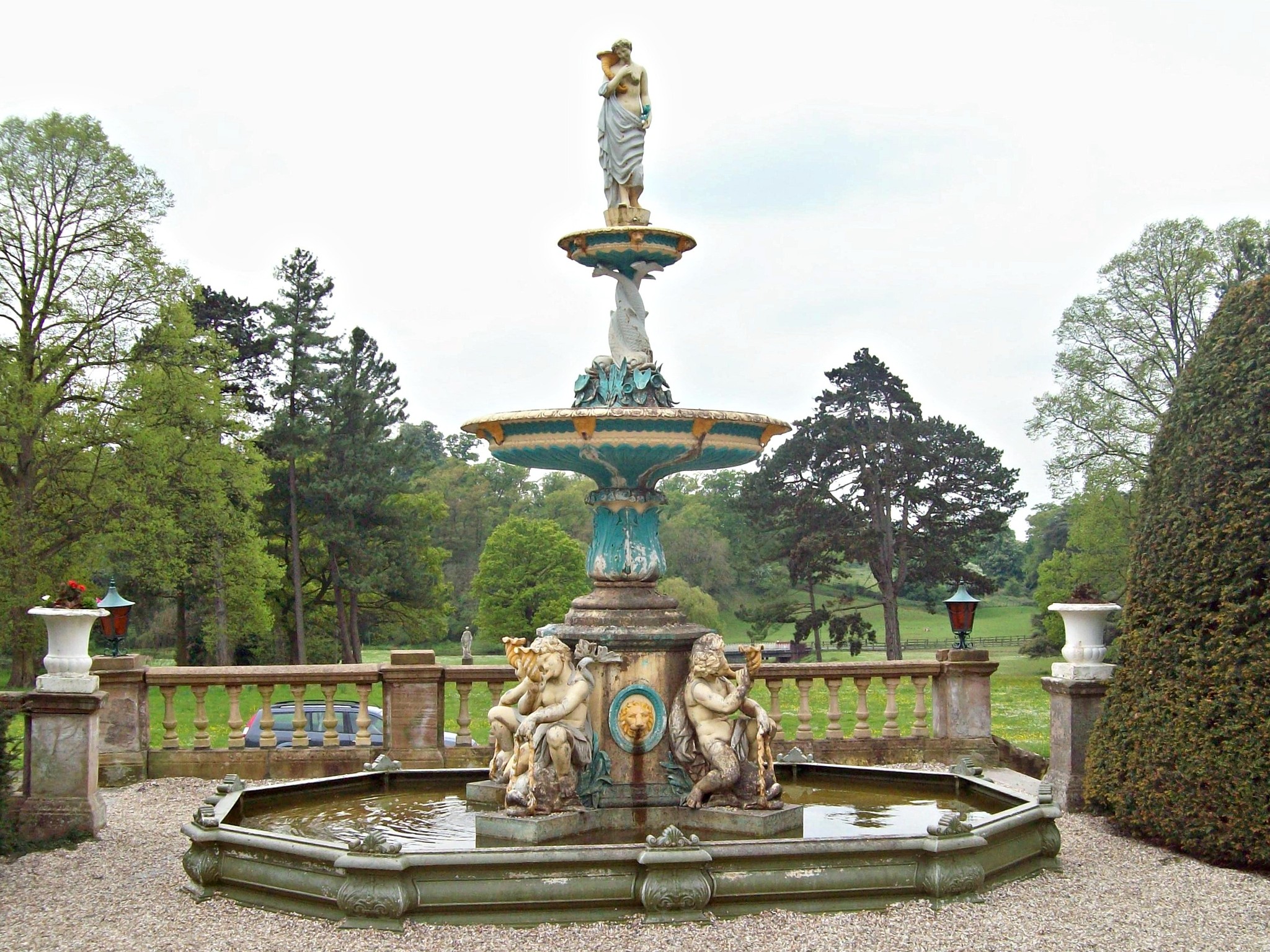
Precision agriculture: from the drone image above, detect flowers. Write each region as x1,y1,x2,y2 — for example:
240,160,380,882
36,580,101,610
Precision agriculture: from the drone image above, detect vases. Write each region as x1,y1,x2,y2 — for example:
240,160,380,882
28,606,111,677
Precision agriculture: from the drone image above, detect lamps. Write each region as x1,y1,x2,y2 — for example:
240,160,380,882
97,576,135,657
942,576,982,649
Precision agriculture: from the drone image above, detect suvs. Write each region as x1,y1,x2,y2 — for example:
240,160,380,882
240,699,479,747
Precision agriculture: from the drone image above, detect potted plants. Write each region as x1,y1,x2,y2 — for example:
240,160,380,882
1047,582,1121,664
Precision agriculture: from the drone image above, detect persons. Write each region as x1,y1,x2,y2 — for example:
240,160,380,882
598,38,652,208
685,632,771,811
488,636,593,798
461,627,473,659
